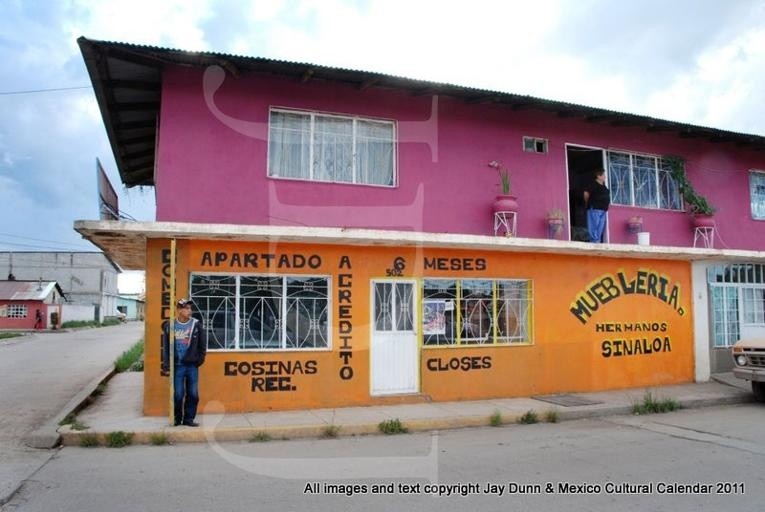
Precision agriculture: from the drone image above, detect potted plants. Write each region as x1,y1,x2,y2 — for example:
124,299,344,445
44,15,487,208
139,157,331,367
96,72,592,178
662,153,719,235
493,167,520,219
628,214,644,232
545,207,565,228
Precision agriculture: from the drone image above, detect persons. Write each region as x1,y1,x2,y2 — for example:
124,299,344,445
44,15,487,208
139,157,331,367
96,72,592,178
33,308,44,332
162,298,206,424
581,168,610,243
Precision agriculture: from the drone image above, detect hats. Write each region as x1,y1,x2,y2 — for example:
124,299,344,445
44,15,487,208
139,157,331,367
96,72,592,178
176,297,192,309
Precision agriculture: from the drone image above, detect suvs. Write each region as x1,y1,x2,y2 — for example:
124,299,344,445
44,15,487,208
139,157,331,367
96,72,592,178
190,309,276,347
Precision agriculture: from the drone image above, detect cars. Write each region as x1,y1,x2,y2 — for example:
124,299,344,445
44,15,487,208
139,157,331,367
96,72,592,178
730,335,765,403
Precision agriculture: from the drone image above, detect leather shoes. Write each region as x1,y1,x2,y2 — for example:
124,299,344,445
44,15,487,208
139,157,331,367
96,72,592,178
183,419,199,427
173,421,182,427
